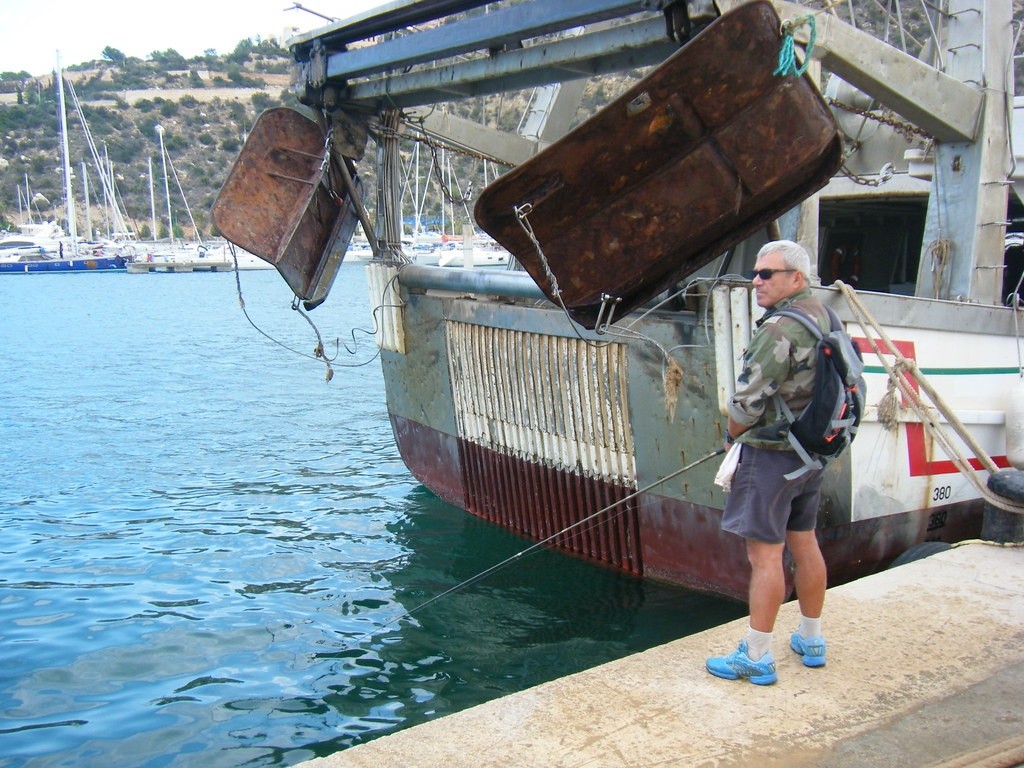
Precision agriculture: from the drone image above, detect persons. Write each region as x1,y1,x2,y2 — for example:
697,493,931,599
706,240,832,686
59,241,63,258
39,246,45,253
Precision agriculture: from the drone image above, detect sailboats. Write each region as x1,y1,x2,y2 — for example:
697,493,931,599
398,133,512,265
0,49,276,275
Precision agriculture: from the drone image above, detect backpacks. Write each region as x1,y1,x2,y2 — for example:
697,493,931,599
760,303,867,481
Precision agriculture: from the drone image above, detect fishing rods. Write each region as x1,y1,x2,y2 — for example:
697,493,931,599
332,448,725,660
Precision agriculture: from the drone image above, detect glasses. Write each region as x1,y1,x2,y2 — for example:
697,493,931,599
751,268,796,280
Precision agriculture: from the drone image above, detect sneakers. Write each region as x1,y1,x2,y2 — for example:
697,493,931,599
705,638,778,685
790,624,827,666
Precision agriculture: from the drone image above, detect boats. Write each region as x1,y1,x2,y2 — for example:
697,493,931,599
210,1,1024,613
